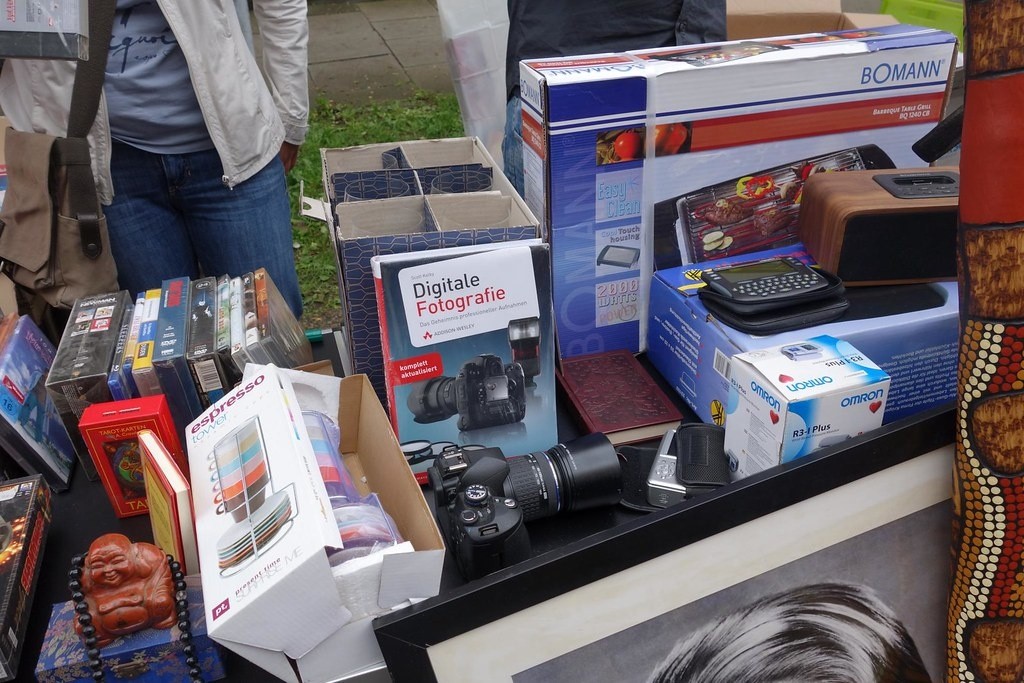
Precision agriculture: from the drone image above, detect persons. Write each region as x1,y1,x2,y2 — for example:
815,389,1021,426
0,0,308,326
73,533,180,649
500,0,729,202
645,579,933,683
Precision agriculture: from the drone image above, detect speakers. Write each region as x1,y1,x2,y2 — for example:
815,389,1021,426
797,166,961,286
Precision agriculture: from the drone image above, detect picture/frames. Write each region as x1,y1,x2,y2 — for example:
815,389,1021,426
371,403,957,683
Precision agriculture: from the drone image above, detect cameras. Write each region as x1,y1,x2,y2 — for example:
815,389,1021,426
407,355,527,433
427,431,624,584
645,427,687,509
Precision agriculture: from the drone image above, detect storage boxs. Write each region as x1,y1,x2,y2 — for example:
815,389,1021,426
726,0,900,42
319,134,542,416
722,334,892,482
78,394,189,519
45,290,135,483
0,473,55,683
186,362,446,683
518,24,960,359
635,244,959,451
35,574,226,683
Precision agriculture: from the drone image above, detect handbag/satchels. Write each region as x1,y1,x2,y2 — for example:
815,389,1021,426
0,126,119,310
